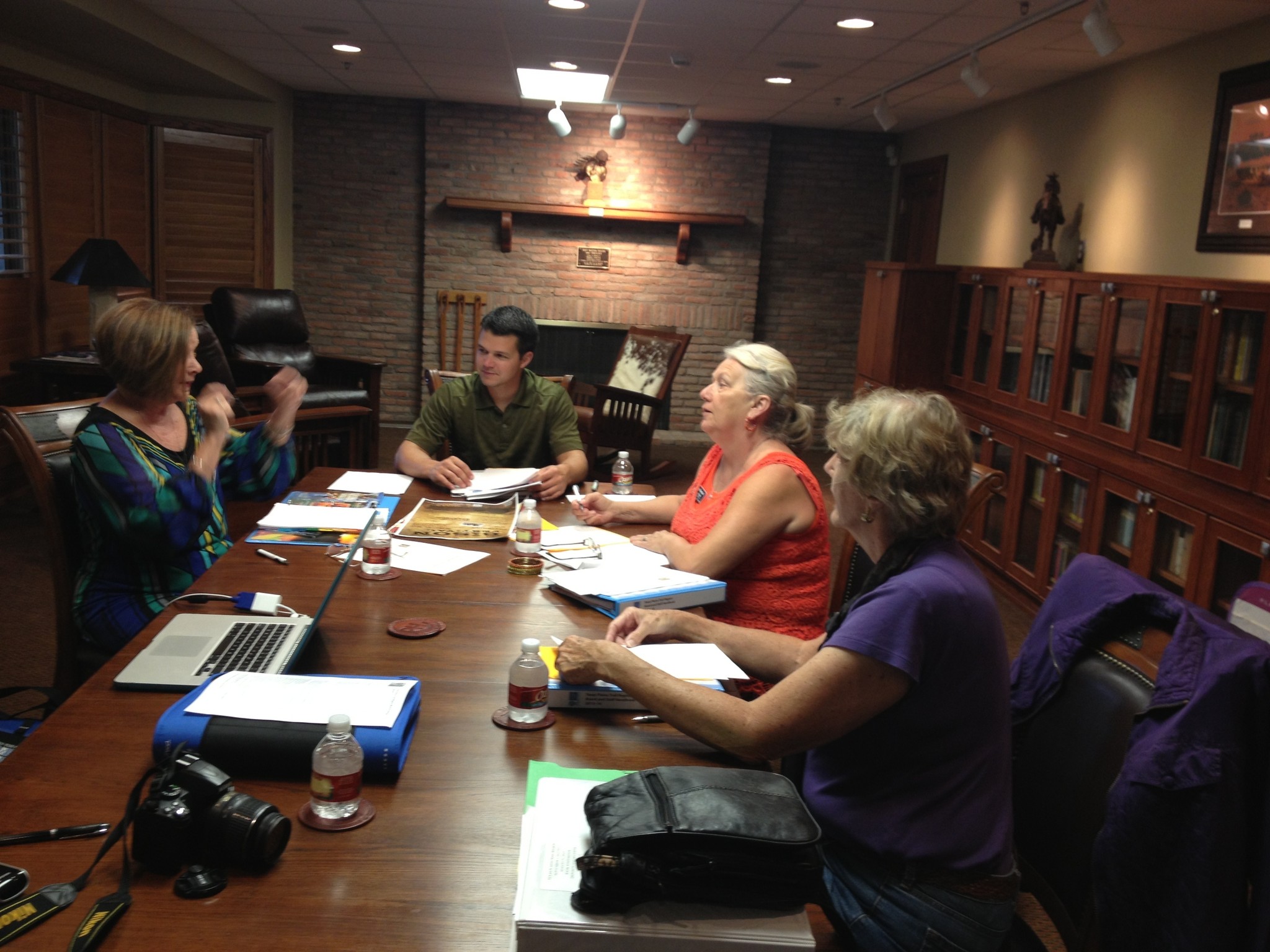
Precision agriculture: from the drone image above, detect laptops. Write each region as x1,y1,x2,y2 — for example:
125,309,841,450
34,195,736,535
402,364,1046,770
112,510,379,690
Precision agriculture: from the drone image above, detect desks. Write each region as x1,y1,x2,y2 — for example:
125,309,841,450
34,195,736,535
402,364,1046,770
0,467,846,952
11,345,110,399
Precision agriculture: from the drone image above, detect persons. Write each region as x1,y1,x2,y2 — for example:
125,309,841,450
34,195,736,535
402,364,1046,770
61,296,306,688
393,303,591,502
571,340,838,703
1031,172,1066,222
553,384,1018,952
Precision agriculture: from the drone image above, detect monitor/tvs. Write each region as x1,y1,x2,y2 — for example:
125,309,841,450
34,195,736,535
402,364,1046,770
529,318,677,429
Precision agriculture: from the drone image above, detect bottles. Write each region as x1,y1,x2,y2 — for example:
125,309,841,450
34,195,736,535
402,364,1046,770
514,498,543,551
509,637,550,722
612,451,634,493
361,513,389,574
310,716,362,822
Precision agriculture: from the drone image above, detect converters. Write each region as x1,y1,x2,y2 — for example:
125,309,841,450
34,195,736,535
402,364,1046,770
167,592,308,618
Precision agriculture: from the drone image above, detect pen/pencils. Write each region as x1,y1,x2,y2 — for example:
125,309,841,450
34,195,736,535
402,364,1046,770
572,484,584,511
629,714,666,724
591,479,600,491
0,822,110,846
256,548,291,564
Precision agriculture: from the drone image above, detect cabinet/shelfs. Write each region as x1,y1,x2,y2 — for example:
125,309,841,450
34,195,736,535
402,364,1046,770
944,266,1270,683
857,260,948,385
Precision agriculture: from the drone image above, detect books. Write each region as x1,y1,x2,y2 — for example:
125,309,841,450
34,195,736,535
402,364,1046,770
1012,328,1261,595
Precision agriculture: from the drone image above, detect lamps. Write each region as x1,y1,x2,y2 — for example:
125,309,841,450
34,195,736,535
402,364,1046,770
49,237,155,354
677,109,701,146
548,100,572,138
609,104,626,139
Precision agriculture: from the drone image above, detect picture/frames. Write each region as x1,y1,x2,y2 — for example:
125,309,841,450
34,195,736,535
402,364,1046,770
1196,61,1270,253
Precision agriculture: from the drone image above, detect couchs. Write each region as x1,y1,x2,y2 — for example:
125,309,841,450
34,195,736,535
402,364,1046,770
193,286,388,470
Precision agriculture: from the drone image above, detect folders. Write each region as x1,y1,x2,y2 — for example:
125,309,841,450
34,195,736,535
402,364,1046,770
536,645,727,716
549,565,726,621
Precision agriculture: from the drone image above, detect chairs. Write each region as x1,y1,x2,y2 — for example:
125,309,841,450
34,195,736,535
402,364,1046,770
424,369,574,462
562,325,692,472
782,458,1007,799
1005,552,1270,952
0,396,110,683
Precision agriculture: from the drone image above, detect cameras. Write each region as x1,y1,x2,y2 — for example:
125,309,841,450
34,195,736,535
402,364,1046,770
127,748,291,874
